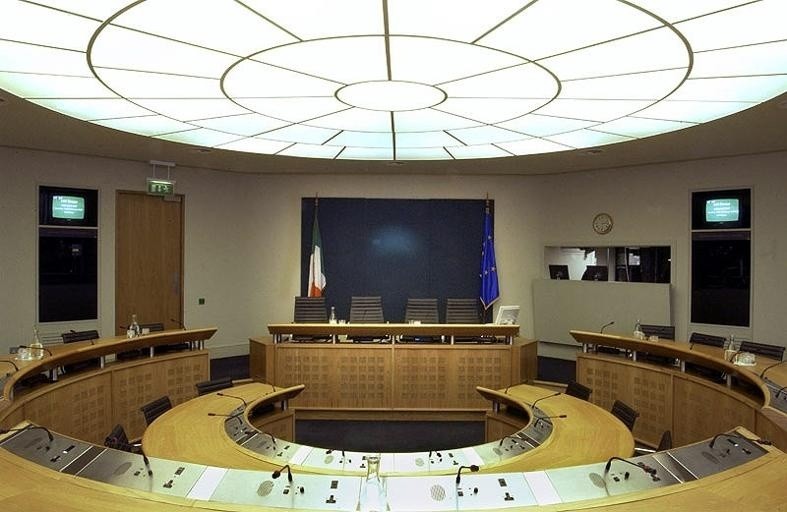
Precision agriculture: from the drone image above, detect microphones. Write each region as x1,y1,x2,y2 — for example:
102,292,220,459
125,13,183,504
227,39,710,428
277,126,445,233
256,375,276,392
170,319,186,330
272,464,293,482
534,415,567,428
531,392,561,409
325,447,345,456
70,329,94,345
504,379,528,394
2,427,54,441
217,393,247,406
646,329,664,341
729,347,761,361
19,344,52,356
689,337,708,350
111,437,153,476
708,433,772,449
760,361,784,378
775,386,785,398
244,431,276,444
208,412,243,425
605,456,656,475
120,326,140,337
499,435,528,446
456,464,479,484
429,448,442,458
601,322,614,334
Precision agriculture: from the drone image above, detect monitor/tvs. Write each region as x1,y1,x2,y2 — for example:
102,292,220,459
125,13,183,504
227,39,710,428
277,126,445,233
586,264,608,281
494,305,521,325
549,264,569,280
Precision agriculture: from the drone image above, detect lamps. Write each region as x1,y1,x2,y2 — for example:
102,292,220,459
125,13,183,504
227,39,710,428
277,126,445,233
130,321,168,357
107,374,232,450
290,294,482,345
638,322,785,359
60,328,104,373
566,377,673,453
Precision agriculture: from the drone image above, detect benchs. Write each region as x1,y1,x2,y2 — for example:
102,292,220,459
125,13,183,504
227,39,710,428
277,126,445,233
307,203,327,297
480,201,501,311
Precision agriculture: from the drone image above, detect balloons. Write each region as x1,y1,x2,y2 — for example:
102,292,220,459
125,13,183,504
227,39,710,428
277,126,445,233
592,213,613,235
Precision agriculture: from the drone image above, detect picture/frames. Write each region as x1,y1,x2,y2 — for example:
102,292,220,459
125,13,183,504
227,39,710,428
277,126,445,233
568,331,786,448
0,326,214,441
248,319,540,425
138,376,636,477
8,422,783,511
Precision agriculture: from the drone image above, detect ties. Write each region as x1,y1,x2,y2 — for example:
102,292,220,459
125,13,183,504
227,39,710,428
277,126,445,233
586,264,608,281
549,264,569,280
494,305,521,325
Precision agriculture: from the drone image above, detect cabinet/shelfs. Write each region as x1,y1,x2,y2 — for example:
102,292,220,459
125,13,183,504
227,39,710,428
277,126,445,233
30,325,45,360
633,318,643,339
328,305,337,325
727,333,737,350
127,314,141,336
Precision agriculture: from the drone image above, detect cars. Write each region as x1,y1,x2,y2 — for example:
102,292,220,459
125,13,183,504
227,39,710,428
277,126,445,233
142,327,150,336
125,328,135,338
17,347,29,360
649,335,658,343
338,318,346,324
